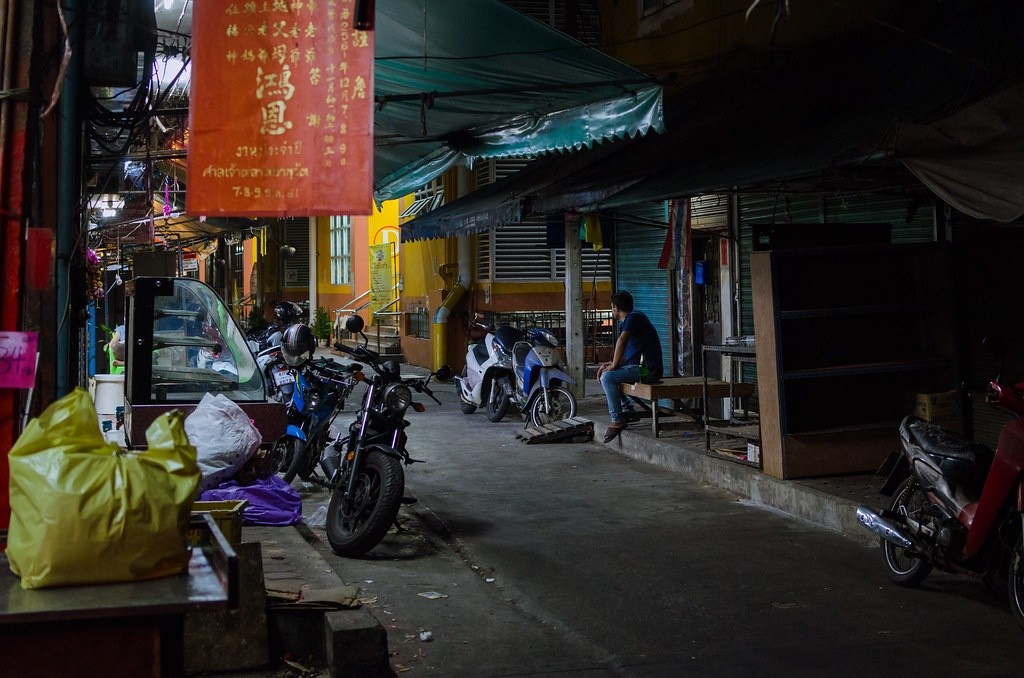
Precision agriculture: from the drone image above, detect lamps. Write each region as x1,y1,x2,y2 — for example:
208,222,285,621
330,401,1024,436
277,241,296,258
215,258,225,268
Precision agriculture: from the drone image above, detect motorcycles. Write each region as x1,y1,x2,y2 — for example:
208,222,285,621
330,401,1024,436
854,374,1024,624
320,316,453,559
510,316,579,428
270,324,364,484
248,301,303,403
454,313,524,423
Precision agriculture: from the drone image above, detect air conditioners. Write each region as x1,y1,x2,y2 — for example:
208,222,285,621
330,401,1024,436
97,140,121,193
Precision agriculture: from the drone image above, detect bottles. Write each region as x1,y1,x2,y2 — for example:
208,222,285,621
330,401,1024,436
638,354,649,384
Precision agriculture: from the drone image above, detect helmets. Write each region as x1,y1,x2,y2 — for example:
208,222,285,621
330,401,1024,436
274,302,304,321
280,324,316,366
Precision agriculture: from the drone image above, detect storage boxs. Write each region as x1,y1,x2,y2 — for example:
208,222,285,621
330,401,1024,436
748,441,760,464
189,499,247,545
911,387,958,423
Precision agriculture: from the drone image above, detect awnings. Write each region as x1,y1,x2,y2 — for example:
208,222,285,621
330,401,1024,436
401,133,681,243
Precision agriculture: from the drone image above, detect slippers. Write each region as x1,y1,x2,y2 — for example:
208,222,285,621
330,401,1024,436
622,412,641,422
603,422,629,443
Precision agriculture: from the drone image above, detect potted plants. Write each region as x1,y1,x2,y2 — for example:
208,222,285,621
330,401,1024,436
310,307,335,348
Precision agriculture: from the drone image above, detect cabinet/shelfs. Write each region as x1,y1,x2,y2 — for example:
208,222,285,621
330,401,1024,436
749,239,972,480
701,345,761,469
152,308,239,403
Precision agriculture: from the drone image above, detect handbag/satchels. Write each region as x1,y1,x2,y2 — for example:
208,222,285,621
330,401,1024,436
5,386,204,592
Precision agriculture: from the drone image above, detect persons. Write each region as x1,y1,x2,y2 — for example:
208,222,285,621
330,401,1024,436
275,302,302,326
247,301,269,330
598,292,663,444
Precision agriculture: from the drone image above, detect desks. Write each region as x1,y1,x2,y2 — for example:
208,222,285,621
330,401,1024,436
0,512,240,678
619,376,754,439
